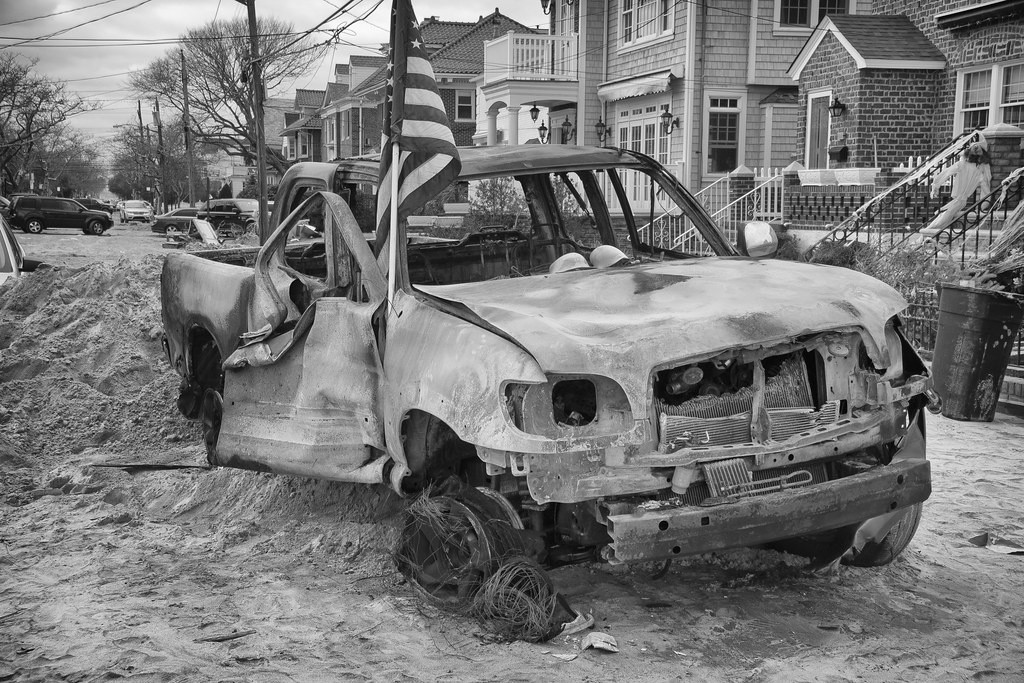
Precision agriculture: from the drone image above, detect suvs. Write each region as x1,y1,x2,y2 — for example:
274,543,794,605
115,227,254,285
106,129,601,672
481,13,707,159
75,196,113,212
196,198,272,239
3,196,114,235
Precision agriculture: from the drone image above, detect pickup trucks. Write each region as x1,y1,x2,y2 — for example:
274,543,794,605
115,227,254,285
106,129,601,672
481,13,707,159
159,145,944,613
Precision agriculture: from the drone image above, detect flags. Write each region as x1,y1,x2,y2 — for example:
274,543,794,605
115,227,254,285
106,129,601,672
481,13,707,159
376,0,462,277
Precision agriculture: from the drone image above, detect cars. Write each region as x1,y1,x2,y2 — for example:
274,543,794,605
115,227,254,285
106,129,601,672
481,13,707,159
1,213,45,286
120,200,153,223
152,209,207,233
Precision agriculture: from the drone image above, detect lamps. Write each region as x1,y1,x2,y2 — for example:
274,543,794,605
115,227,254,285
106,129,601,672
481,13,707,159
661,106,678,134
541,0,554,15
827,97,846,117
561,114,577,141
594,116,611,142
537,122,551,144
529,101,540,123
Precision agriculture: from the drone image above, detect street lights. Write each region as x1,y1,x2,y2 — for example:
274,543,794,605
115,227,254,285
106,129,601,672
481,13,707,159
114,123,168,214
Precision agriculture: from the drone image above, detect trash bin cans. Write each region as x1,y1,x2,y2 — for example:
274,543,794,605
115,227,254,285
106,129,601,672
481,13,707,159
924,280,1023,423
